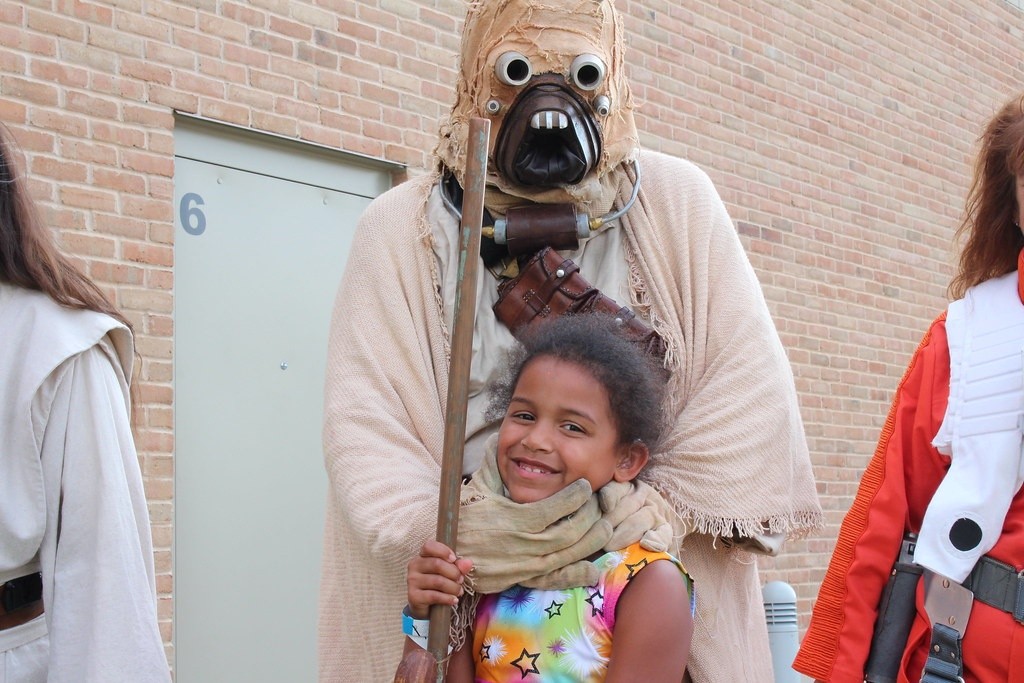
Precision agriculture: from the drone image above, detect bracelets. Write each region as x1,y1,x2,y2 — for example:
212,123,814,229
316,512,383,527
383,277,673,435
402,604,453,656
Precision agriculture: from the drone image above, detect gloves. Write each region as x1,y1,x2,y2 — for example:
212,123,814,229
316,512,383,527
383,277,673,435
600,477,675,557
452,433,616,587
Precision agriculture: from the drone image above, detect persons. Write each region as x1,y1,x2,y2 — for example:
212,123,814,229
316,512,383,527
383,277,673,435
791,97,1024,682
320,0,822,683
0,122,175,683
397,309,698,683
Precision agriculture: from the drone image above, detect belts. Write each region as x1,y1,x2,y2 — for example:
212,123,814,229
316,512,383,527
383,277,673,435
0,572,45,630
896,533,1024,625
